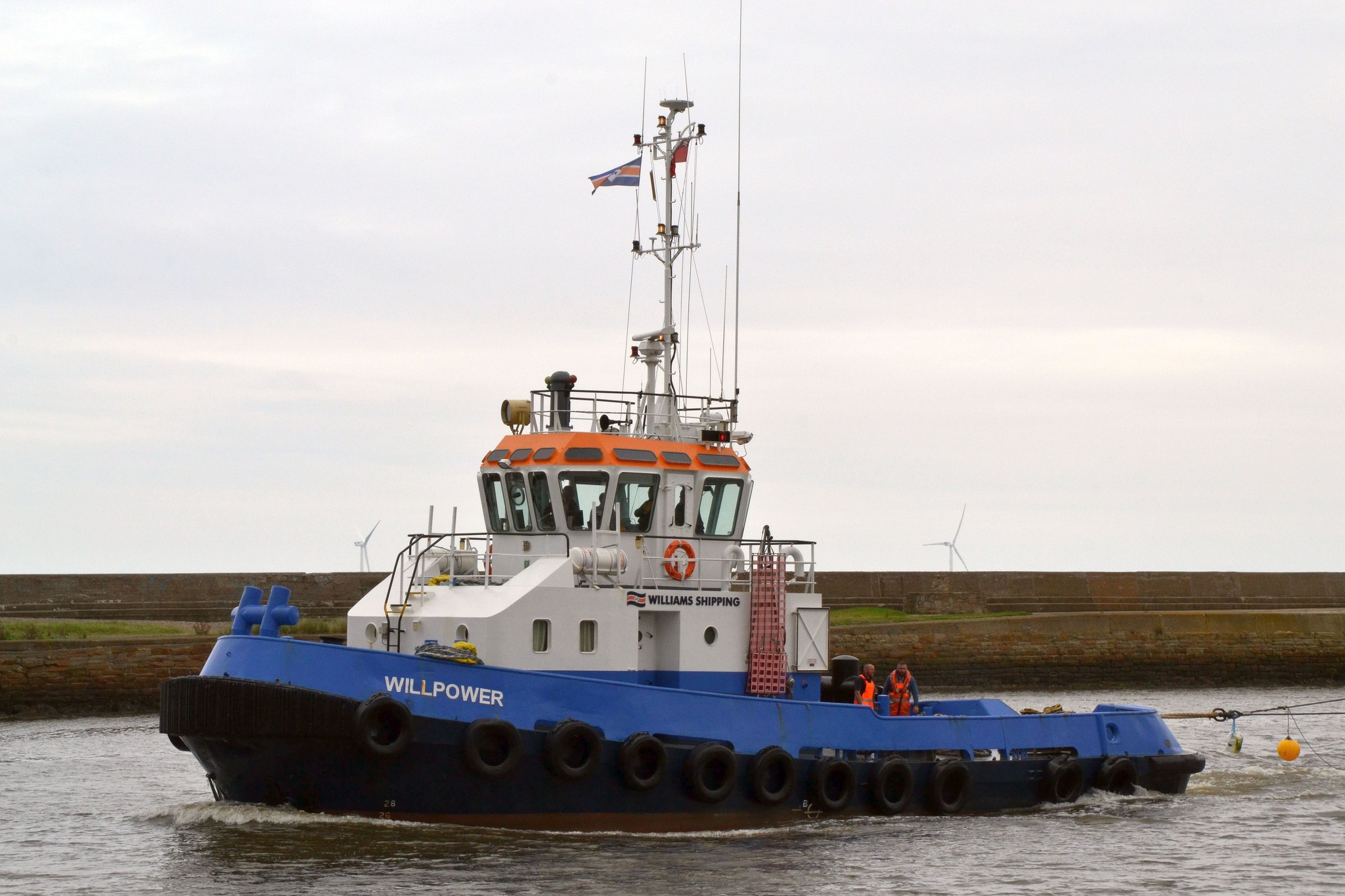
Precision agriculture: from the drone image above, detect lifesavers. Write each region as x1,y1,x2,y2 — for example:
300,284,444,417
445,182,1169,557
1097,754,1138,795
871,756,915,815
809,757,857,813
750,746,797,806
615,734,669,792
684,744,737,803
463,718,524,779
1044,755,1084,803
664,540,696,581
928,756,972,816
483,544,492,575
545,720,602,781
352,695,414,758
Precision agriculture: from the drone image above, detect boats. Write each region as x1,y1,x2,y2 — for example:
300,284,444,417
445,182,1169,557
152,5,1208,821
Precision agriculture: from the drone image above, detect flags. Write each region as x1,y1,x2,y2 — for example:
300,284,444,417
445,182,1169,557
671,138,690,177
588,155,642,196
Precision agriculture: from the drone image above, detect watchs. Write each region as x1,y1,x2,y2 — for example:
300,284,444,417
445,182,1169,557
914,704,918,707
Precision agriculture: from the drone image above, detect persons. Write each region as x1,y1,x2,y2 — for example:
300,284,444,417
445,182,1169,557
675,490,705,534
853,663,876,710
542,485,580,530
634,487,654,532
881,661,919,718
588,492,617,531
